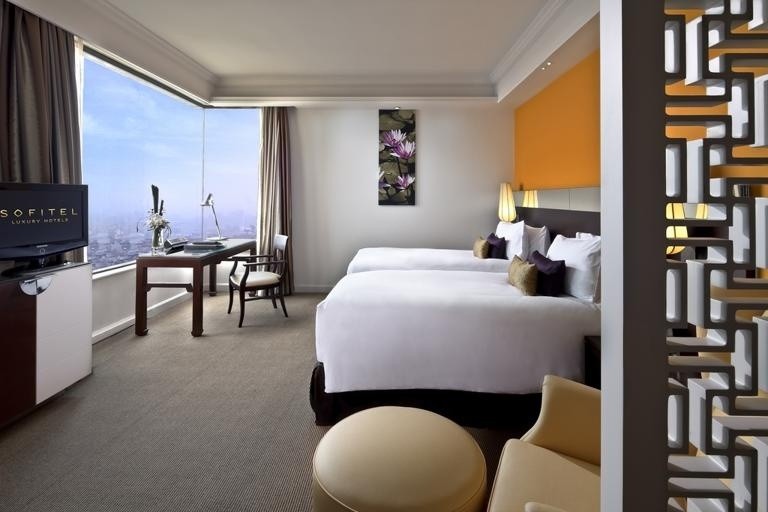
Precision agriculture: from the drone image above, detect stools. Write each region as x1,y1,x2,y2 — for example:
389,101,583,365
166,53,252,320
309,406,487,512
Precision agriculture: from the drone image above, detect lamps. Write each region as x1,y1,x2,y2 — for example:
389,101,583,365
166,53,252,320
200,194,228,242
661,203,706,269
498,182,516,224
522,190,539,208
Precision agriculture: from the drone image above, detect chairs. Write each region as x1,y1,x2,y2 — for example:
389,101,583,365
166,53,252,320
228,234,289,328
486,266,767,512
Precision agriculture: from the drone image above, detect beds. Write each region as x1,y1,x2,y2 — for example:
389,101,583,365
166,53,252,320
346,248,507,272
309,272,601,432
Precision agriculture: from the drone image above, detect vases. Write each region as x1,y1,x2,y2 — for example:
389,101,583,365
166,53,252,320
150,228,164,256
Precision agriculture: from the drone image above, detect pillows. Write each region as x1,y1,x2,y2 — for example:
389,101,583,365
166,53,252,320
473,221,601,305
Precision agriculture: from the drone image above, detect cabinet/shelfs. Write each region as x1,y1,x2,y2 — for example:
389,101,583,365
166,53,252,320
0,263,94,427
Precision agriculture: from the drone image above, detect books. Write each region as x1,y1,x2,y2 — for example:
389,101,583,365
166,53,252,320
183,241,224,250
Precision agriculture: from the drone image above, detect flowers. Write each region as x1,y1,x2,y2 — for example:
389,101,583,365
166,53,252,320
136,184,172,246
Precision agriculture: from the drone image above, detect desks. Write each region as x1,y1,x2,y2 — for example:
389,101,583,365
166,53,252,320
136,239,258,339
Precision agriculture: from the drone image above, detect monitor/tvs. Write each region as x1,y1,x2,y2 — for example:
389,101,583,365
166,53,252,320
0,183,88,275
